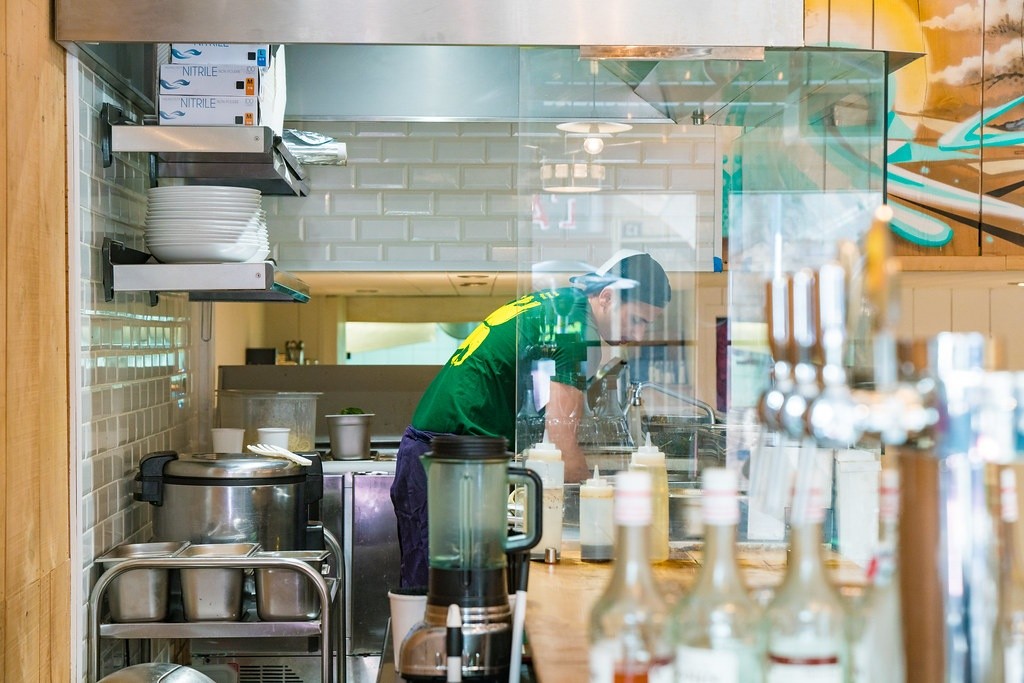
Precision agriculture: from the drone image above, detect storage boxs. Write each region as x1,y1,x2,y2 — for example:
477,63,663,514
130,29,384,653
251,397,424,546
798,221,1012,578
213,388,324,451
157,43,272,125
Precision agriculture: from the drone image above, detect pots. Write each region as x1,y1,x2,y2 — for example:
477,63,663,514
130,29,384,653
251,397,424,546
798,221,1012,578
132,450,308,552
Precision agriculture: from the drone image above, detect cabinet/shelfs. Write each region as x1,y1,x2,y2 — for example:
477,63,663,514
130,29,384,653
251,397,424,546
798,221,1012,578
86,525,345,683
97,101,312,307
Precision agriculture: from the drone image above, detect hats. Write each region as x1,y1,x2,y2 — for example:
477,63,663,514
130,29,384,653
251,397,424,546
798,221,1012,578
570,253,669,308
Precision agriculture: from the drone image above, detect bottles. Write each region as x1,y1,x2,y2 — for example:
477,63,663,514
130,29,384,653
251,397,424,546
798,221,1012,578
588,470,674,683
579,464,614,564
629,432,670,564
760,463,902,683
667,466,765,683
525,426,565,563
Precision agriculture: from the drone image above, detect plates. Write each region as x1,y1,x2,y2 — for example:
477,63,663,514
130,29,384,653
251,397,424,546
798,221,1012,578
142,185,270,263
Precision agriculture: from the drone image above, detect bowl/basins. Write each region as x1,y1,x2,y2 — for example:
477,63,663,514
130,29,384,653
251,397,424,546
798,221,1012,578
256,427,291,450
209,428,246,454
324,413,376,460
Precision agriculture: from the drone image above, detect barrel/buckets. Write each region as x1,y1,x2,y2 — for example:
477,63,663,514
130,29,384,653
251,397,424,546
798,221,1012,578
212,387,324,452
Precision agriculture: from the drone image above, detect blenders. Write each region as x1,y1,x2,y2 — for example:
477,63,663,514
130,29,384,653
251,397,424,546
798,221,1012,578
399,435,543,683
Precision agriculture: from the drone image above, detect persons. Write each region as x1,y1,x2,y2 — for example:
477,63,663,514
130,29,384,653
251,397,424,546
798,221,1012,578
390,248,671,595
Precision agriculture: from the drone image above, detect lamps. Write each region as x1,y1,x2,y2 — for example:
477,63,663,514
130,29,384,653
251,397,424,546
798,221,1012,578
555,58,633,154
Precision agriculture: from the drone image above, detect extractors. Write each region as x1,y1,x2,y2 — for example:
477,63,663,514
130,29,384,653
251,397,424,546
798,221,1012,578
101,236,311,306
